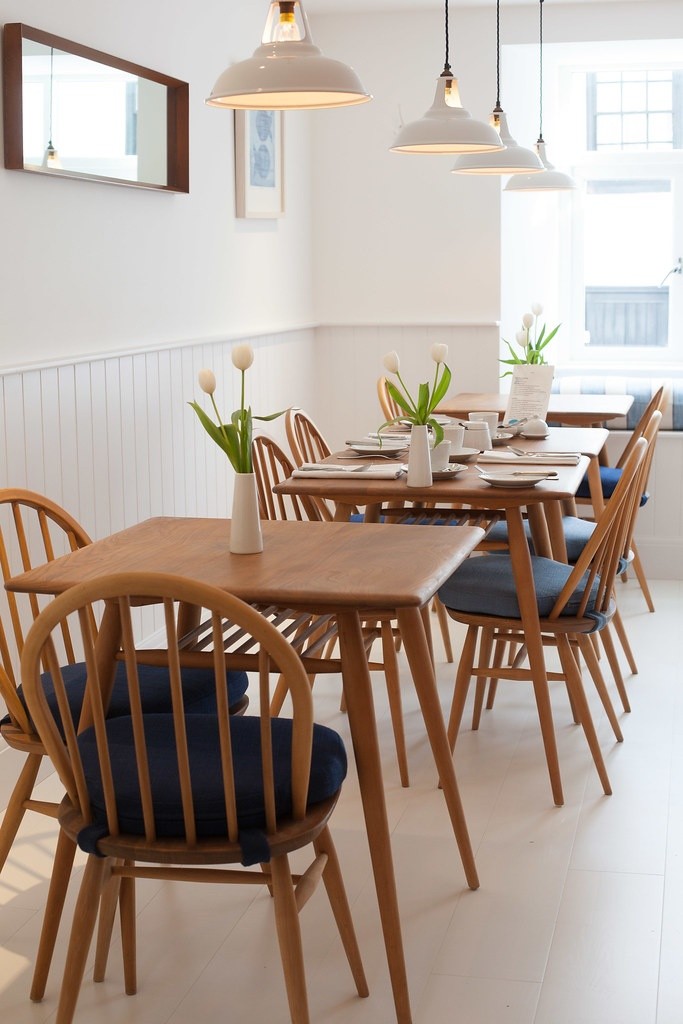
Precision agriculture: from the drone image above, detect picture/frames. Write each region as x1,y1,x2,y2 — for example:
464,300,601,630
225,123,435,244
233,110,284,218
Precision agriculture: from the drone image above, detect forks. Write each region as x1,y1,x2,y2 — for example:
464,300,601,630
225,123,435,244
337,452,410,460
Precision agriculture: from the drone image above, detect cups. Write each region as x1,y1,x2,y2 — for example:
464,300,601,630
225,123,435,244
468,411,499,439
429,439,451,471
431,425,465,455
463,420,492,452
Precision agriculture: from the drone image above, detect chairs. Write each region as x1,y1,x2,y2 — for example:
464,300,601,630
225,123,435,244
0,379,671,1024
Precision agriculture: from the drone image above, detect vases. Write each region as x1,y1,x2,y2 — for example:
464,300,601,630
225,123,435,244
227,472,263,554
407,425,434,489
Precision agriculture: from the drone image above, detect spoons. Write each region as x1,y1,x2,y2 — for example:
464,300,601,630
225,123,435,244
302,463,374,471
507,445,580,456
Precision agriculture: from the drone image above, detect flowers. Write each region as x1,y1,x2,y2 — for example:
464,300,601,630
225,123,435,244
187,343,301,474
498,303,563,381
374,342,456,449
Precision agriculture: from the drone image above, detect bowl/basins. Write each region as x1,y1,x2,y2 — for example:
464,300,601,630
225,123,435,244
520,414,550,440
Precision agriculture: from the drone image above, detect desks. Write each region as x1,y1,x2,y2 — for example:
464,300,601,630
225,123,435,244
431,392,636,577
341,427,638,662
4,517,484,1024
270,453,594,803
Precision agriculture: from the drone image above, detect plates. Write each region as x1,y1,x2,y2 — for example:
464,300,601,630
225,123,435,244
400,418,451,428
400,463,468,480
491,433,513,447
349,443,408,457
450,448,481,462
478,470,548,488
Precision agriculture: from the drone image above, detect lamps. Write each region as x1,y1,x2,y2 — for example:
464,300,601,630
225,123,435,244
43,47,59,168
204,0,372,111
503,0,578,192
390,0,506,155
450,0,548,174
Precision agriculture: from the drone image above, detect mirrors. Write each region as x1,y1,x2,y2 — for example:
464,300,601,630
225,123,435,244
2,22,193,194
